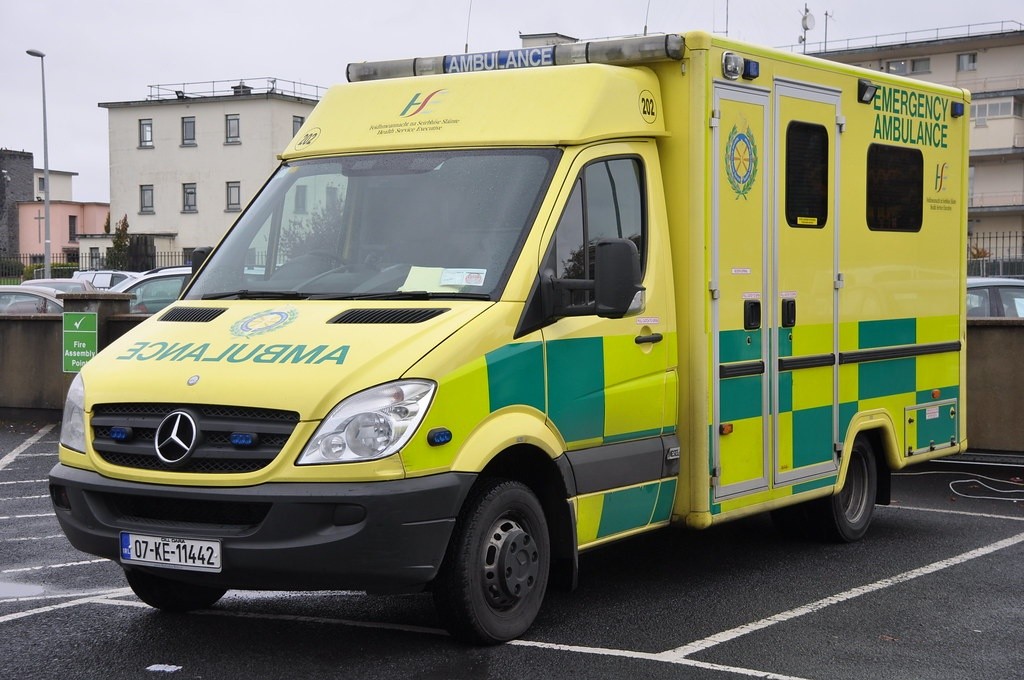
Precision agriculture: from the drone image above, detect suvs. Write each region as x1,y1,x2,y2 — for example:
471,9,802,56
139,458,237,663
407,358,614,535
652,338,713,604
104,262,283,315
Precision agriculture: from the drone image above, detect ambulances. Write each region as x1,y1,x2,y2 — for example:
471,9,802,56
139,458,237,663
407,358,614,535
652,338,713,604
45,30,969,649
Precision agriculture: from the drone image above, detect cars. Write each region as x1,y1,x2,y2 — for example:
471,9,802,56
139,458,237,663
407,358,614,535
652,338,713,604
965,274,1024,320
69,268,144,292
0,285,66,314
6,278,99,312
